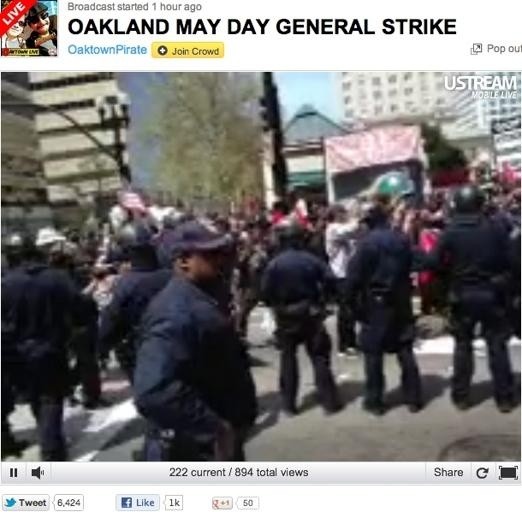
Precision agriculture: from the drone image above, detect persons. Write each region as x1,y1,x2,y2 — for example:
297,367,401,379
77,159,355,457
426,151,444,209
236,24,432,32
1,14,26,49
1,158,521,461
26,2,57,56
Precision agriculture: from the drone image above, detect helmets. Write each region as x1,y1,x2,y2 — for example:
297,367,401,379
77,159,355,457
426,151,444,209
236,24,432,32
452,185,489,213
114,220,157,250
272,217,303,237
1,232,33,248
359,202,385,220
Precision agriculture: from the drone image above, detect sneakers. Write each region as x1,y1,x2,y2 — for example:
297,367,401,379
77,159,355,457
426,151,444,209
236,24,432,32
282,396,521,415
82,398,112,408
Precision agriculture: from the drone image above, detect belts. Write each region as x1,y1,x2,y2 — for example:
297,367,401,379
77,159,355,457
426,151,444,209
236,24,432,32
148,429,187,441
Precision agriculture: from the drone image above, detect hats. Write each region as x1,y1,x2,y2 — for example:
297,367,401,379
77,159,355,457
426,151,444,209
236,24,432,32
164,225,232,256
36,228,68,249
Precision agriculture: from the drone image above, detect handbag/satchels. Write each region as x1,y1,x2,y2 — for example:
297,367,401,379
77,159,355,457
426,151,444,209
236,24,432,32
451,283,507,322
355,286,407,331
279,300,324,345
